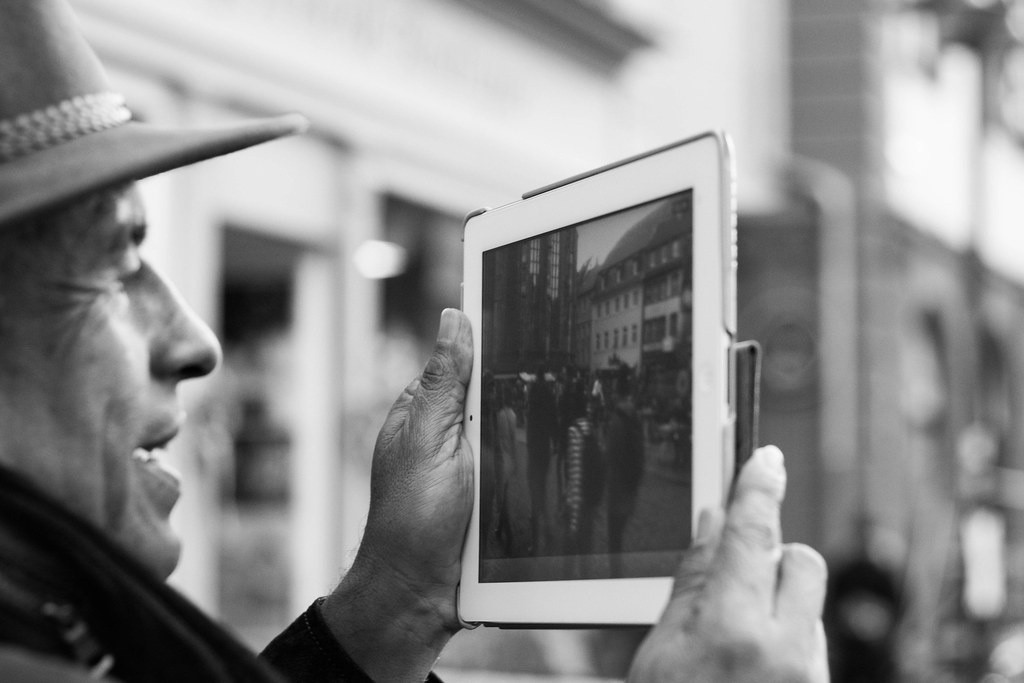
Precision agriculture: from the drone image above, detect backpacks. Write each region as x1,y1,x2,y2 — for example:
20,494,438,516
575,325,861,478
583,436,606,506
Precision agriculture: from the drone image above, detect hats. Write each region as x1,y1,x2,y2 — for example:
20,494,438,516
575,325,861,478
1,0,293,227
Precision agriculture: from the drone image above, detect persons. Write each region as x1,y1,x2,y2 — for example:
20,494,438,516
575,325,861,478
479,358,694,584
2,0,836,683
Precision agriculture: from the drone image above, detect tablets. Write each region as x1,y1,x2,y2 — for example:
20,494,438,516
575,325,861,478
451,128,742,631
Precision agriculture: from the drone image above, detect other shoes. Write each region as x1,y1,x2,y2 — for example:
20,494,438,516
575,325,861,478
492,527,556,558
